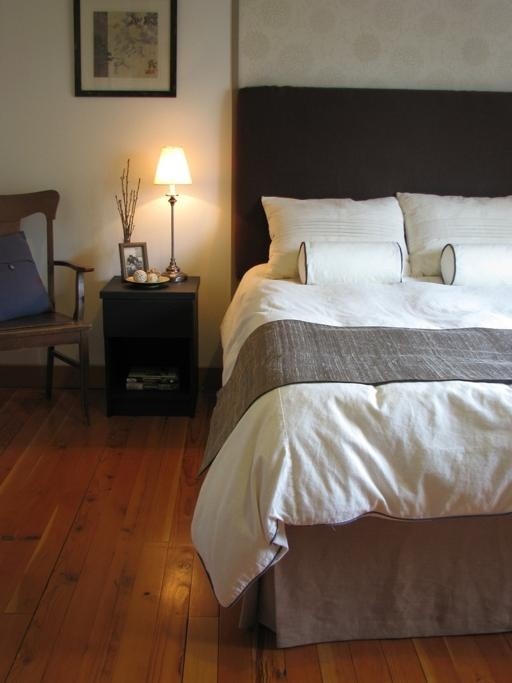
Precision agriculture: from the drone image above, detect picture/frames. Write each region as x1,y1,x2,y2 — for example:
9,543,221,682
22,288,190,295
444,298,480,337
71,0,177,98
118,240,148,283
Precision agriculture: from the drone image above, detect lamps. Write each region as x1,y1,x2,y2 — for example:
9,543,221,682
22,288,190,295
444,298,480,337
152,145,192,283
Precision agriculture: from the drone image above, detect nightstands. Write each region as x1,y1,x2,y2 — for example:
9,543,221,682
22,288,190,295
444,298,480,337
99,275,201,418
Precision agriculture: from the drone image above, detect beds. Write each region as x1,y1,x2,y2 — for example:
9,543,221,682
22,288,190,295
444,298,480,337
231,84,510,649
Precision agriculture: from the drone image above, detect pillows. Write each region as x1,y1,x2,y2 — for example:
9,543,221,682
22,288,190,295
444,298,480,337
0,231,53,322
259,190,511,286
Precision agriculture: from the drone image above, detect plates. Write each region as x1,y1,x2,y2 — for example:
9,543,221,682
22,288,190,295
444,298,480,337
125,275,170,287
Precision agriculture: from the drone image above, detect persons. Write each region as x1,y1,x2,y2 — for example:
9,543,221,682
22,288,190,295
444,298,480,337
125,254,142,275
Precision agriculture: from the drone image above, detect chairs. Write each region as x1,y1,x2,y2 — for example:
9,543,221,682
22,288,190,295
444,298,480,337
0,187,94,429
